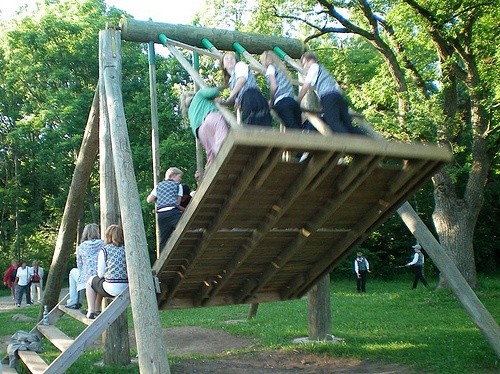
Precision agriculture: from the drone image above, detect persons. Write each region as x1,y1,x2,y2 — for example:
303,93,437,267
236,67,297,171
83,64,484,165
14,260,33,307
146,167,186,256
180,87,229,170
194,171,202,188
85,224,129,319
406,244,430,289
215,53,273,127
259,50,303,131
29,260,44,304
3,259,21,305
297,51,356,135
181,185,192,205
354,251,372,293
66,223,104,309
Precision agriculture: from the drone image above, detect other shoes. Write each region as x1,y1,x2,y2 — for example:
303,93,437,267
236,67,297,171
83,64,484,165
81,310,101,317
77,303,81,309
66,304,77,309
85,313,95,319
